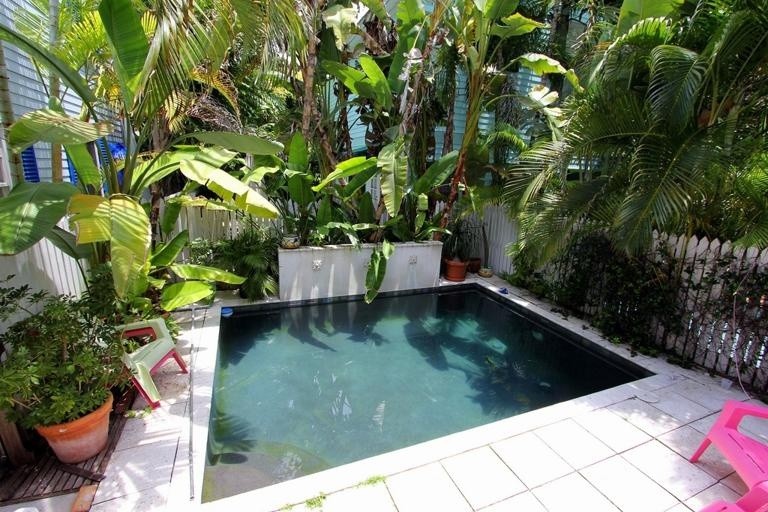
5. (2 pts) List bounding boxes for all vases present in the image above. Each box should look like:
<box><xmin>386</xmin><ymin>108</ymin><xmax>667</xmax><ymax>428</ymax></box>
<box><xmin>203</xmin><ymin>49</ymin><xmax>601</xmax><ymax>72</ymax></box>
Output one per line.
<box><xmin>280</xmin><ymin>234</ymin><xmax>301</xmax><ymax>248</ymax></box>
<box><xmin>468</xmin><ymin>256</ymin><xmax>482</xmax><ymax>274</ymax></box>
<box><xmin>478</xmin><ymin>267</ymin><xmax>493</xmax><ymax>278</ymax></box>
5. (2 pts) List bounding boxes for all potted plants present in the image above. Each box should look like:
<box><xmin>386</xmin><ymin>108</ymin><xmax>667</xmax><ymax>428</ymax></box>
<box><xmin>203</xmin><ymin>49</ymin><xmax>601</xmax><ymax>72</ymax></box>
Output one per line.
<box><xmin>0</xmin><ymin>272</ymin><xmax>150</xmax><ymax>465</ymax></box>
<box><xmin>214</xmin><ymin>243</ymin><xmax>278</xmax><ymax>302</ymax></box>
<box><xmin>445</xmin><ymin>218</ymin><xmax>478</xmax><ymax>282</ymax></box>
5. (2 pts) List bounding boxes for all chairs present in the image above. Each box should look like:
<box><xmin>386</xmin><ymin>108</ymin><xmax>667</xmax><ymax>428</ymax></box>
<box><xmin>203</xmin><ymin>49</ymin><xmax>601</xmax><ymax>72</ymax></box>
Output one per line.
<box><xmin>75</xmin><ymin>316</ymin><xmax>189</xmax><ymax>412</ymax></box>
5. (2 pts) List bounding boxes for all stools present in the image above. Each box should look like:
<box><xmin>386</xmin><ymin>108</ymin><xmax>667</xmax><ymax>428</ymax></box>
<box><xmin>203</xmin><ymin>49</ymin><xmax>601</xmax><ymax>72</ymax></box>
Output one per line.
<box><xmin>698</xmin><ymin>480</ymin><xmax>768</xmax><ymax>511</ymax></box>
<box><xmin>691</xmin><ymin>399</ymin><xmax>767</xmax><ymax>490</ymax></box>
